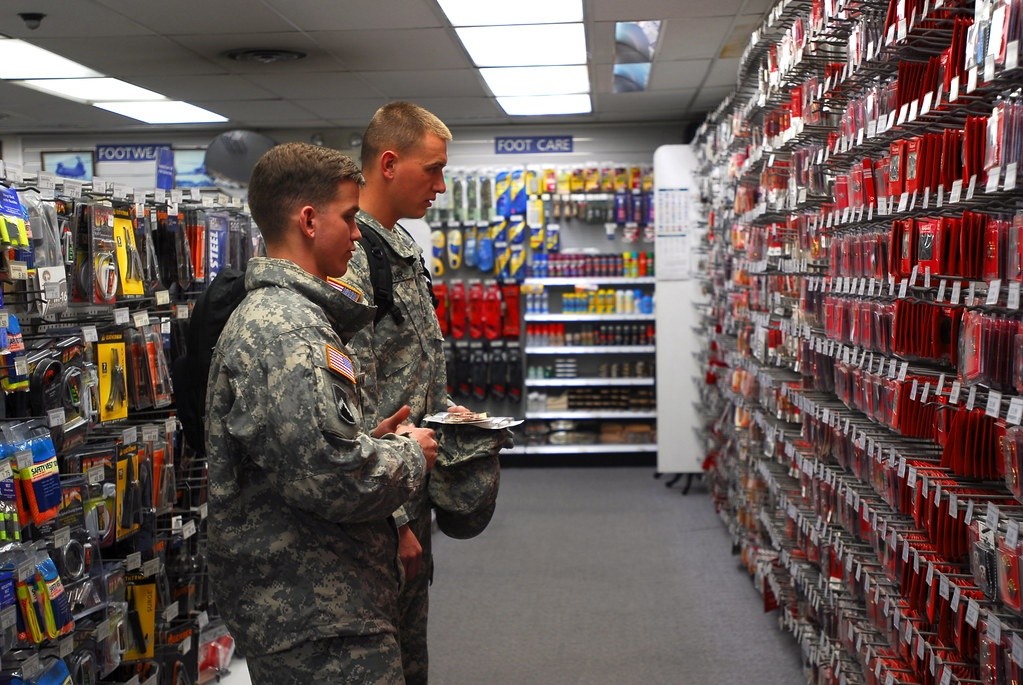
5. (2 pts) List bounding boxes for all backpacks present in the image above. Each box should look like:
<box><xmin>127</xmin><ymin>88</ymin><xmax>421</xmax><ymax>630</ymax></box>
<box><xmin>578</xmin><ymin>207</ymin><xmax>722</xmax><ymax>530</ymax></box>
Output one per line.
<box><xmin>172</xmin><ymin>221</ymin><xmax>440</xmax><ymax>459</ymax></box>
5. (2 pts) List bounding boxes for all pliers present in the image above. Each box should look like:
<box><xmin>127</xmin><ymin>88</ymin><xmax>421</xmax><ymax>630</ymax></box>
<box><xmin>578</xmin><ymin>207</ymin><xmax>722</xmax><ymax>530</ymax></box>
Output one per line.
<box><xmin>180</xmin><ymin>223</ymin><xmax>194</xmax><ymax>279</ymax></box>
<box><xmin>122</xmin><ymin>456</ymin><xmax>141</xmax><ymax>529</ymax></box>
<box><xmin>123</xmin><ymin>226</ymin><xmax>145</xmax><ymax>281</ymax></box>
<box><xmin>105</xmin><ymin>349</ymin><xmax>128</xmax><ymax>411</ymax></box>
<box><xmin>152</xmin><ymin>334</ymin><xmax>175</xmax><ymax>394</ymax></box>
<box><xmin>121</xmin><ymin>584</ymin><xmax>147</xmax><ymax>654</ymax></box>
<box><xmin>136</xmin><ymin>340</ymin><xmax>150</xmax><ymax>397</ymax></box>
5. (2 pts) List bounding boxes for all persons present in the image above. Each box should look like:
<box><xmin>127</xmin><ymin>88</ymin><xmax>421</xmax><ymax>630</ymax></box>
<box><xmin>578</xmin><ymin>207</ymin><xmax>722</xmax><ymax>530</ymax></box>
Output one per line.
<box><xmin>325</xmin><ymin>100</ymin><xmax>478</xmax><ymax>684</ymax></box>
<box><xmin>203</xmin><ymin>144</ymin><xmax>442</xmax><ymax>684</ymax></box>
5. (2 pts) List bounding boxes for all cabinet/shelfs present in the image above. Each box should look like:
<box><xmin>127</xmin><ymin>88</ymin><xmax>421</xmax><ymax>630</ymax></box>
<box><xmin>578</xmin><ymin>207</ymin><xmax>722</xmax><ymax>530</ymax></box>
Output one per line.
<box><xmin>691</xmin><ymin>0</ymin><xmax>1023</xmax><ymax>685</ymax></box>
<box><xmin>0</xmin><ymin>165</ymin><xmax>658</xmax><ymax>685</ymax></box>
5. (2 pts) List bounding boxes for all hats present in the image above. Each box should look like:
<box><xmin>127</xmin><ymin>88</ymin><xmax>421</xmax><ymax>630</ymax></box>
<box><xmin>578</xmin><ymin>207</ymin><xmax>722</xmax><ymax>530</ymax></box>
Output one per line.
<box><xmin>427</xmin><ymin>425</ymin><xmax>500</xmax><ymax>539</ymax></box>
